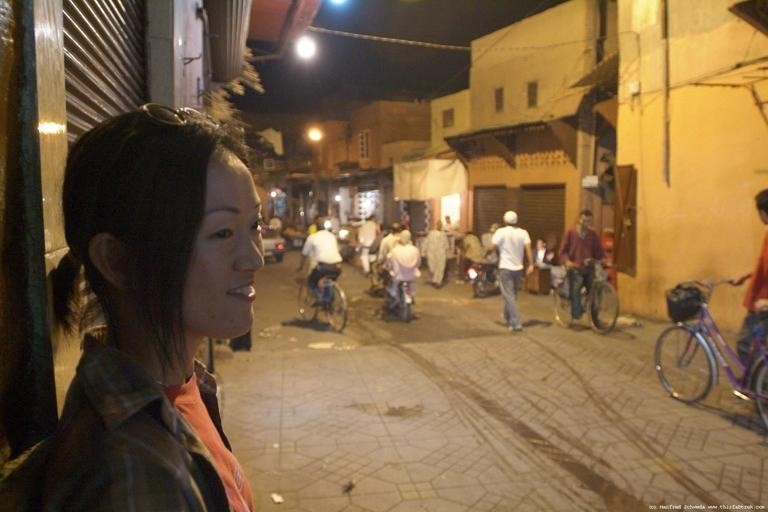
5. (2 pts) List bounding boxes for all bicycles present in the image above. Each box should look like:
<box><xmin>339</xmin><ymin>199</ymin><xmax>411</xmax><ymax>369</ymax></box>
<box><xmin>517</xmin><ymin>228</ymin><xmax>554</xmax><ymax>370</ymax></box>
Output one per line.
<box><xmin>553</xmin><ymin>257</ymin><xmax>619</xmax><ymax>334</ymax></box>
<box><xmin>297</xmin><ymin>267</ymin><xmax>348</xmax><ymax>333</ymax></box>
<box><xmin>369</xmin><ymin>262</ymin><xmax>412</xmax><ymax>321</ymax></box>
<box><xmin>654</xmin><ymin>277</ymin><xmax>767</xmax><ymax>433</ymax></box>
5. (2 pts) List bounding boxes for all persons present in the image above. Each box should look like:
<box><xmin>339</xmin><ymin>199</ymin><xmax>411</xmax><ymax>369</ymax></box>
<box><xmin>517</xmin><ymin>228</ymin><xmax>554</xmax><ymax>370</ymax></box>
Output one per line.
<box><xmin>30</xmin><ymin>103</ymin><xmax>268</xmax><ymax>510</ymax></box>
<box><xmin>294</xmin><ymin>209</ymin><xmax>550</xmax><ymax>332</ymax></box>
<box><xmin>727</xmin><ymin>188</ymin><xmax>767</xmax><ymax>397</ymax></box>
<box><xmin>559</xmin><ymin>208</ymin><xmax>605</xmax><ymax>326</ymax></box>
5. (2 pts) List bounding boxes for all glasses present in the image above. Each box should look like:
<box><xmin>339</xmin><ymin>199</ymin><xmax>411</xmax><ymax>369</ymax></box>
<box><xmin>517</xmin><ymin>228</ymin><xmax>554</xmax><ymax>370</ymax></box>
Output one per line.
<box><xmin>127</xmin><ymin>102</ymin><xmax>202</xmax><ymax>129</ymax></box>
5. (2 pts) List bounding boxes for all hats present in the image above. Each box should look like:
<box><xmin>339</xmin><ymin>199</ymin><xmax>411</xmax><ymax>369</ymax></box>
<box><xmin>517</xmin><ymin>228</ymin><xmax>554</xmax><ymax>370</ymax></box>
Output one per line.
<box><xmin>503</xmin><ymin>212</ymin><xmax>517</xmax><ymax>224</ymax></box>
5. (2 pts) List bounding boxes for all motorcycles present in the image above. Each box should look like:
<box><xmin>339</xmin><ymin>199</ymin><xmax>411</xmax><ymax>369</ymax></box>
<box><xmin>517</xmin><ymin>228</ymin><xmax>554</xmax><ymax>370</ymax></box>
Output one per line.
<box><xmin>469</xmin><ymin>261</ymin><xmax>500</xmax><ymax>299</ymax></box>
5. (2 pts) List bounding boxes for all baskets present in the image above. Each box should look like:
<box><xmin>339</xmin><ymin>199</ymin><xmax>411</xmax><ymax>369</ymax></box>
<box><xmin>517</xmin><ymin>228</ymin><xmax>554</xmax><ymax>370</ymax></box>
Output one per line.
<box><xmin>666</xmin><ymin>287</ymin><xmax>703</xmax><ymax>322</ymax></box>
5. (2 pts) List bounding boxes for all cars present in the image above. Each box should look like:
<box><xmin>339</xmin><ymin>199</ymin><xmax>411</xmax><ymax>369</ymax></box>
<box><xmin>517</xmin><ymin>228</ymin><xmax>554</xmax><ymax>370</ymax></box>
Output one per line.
<box><xmin>259</xmin><ymin>224</ymin><xmax>286</xmax><ymax>263</ymax></box>
